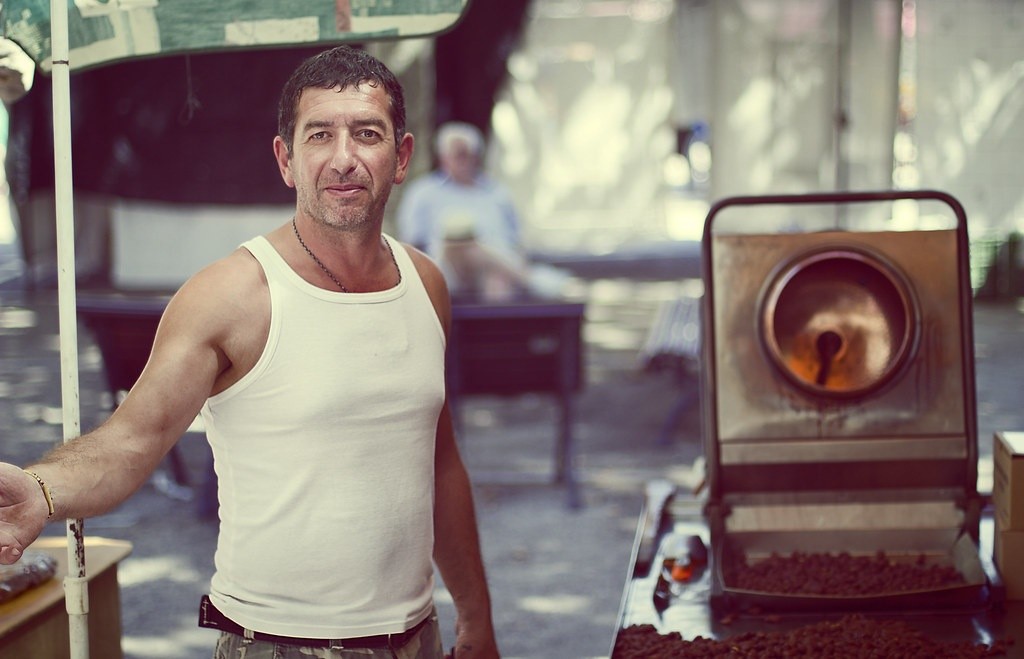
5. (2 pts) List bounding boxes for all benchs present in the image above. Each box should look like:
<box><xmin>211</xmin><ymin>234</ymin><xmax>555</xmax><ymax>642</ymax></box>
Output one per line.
<box><xmin>638</xmin><ymin>297</ymin><xmax>703</xmax><ymax>447</ymax></box>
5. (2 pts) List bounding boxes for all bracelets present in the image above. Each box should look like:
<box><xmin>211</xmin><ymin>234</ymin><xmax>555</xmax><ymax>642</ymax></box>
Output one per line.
<box><xmin>23</xmin><ymin>470</ymin><xmax>55</xmax><ymax>520</ymax></box>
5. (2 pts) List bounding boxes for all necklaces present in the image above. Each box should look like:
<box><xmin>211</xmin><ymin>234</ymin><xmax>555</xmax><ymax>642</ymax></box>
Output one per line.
<box><xmin>293</xmin><ymin>215</ymin><xmax>402</xmax><ymax>292</ymax></box>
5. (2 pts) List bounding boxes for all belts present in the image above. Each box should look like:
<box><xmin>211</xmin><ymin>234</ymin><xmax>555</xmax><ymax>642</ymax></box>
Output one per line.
<box><xmin>199</xmin><ymin>594</ymin><xmax>430</xmax><ymax>650</ymax></box>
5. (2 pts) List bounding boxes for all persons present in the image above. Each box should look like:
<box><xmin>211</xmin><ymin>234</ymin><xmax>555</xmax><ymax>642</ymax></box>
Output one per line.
<box><xmin>0</xmin><ymin>43</ymin><xmax>504</xmax><ymax>659</ymax></box>
<box><xmin>395</xmin><ymin>121</ymin><xmax>524</xmax><ymax>302</ymax></box>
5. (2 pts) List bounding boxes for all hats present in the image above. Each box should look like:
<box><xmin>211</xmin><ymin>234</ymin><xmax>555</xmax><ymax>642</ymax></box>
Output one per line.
<box><xmin>438</xmin><ymin>123</ymin><xmax>483</xmax><ymax>155</ymax></box>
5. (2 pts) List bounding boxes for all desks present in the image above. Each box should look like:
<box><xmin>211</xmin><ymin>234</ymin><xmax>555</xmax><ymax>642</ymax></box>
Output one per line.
<box><xmin>74</xmin><ymin>291</ymin><xmax>588</xmax><ymax>512</ymax></box>
<box><xmin>0</xmin><ymin>535</ymin><xmax>133</xmax><ymax>659</ymax></box>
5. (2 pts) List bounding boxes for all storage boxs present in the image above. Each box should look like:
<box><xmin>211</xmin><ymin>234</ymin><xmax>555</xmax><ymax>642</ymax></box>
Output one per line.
<box><xmin>992</xmin><ymin>431</ymin><xmax>1024</xmax><ymax>603</ymax></box>
